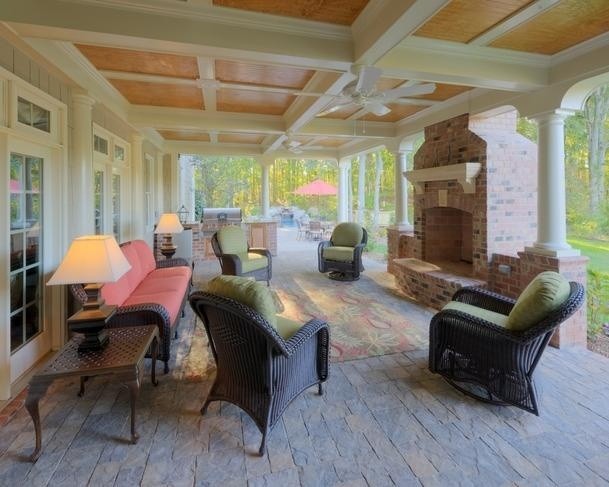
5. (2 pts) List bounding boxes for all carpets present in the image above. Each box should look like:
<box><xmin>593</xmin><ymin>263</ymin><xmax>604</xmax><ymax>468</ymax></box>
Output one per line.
<box><xmin>171</xmin><ymin>272</ymin><xmax>431</xmax><ymax>384</ymax></box>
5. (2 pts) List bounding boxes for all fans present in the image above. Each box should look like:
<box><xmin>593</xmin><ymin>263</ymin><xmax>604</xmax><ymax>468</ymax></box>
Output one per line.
<box><xmin>293</xmin><ymin>64</ymin><xmax>437</xmax><ymax>118</ymax></box>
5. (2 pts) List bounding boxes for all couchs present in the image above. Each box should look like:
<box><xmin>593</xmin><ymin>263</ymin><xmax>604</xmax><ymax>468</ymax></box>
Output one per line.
<box><xmin>68</xmin><ymin>239</ymin><xmax>195</xmax><ymax>375</ymax></box>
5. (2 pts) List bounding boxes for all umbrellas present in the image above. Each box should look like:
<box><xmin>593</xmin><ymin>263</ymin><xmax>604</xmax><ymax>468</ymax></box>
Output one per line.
<box><xmin>291</xmin><ymin>177</ymin><xmax>338</xmax><ymax>221</ymax></box>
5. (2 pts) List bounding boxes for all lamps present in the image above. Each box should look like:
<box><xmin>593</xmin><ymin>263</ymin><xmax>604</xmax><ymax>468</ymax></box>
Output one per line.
<box><xmin>153</xmin><ymin>214</ymin><xmax>184</xmax><ymax>250</ymax></box>
<box><xmin>45</xmin><ymin>234</ymin><xmax>133</xmax><ymax>354</ymax></box>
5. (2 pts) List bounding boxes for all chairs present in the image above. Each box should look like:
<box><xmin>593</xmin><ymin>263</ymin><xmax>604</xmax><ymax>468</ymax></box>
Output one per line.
<box><xmin>295</xmin><ymin>218</ymin><xmax>310</xmax><ymax>241</ymax></box>
<box><xmin>308</xmin><ymin>221</ymin><xmax>322</xmax><ymax>241</ymax></box>
<box><xmin>317</xmin><ymin>222</ymin><xmax>369</xmax><ymax>282</ymax></box>
<box><xmin>186</xmin><ymin>274</ymin><xmax>331</xmax><ymax>458</ymax></box>
<box><xmin>210</xmin><ymin>223</ymin><xmax>273</xmax><ymax>286</ymax></box>
<box><xmin>425</xmin><ymin>271</ymin><xmax>586</xmax><ymax>419</ymax></box>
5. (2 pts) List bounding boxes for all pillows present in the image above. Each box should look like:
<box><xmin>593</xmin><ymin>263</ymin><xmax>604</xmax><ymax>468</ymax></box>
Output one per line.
<box><xmin>506</xmin><ymin>270</ymin><xmax>571</xmax><ymax>331</ymax></box>
<box><xmin>205</xmin><ymin>272</ymin><xmax>279</xmax><ymax>336</ymax></box>
<box><xmin>216</xmin><ymin>224</ymin><xmax>249</xmax><ymax>254</ymax></box>
<box><xmin>331</xmin><ymin>222</ymin><xmax>364</xmax><ymax>247</ymax></box>
